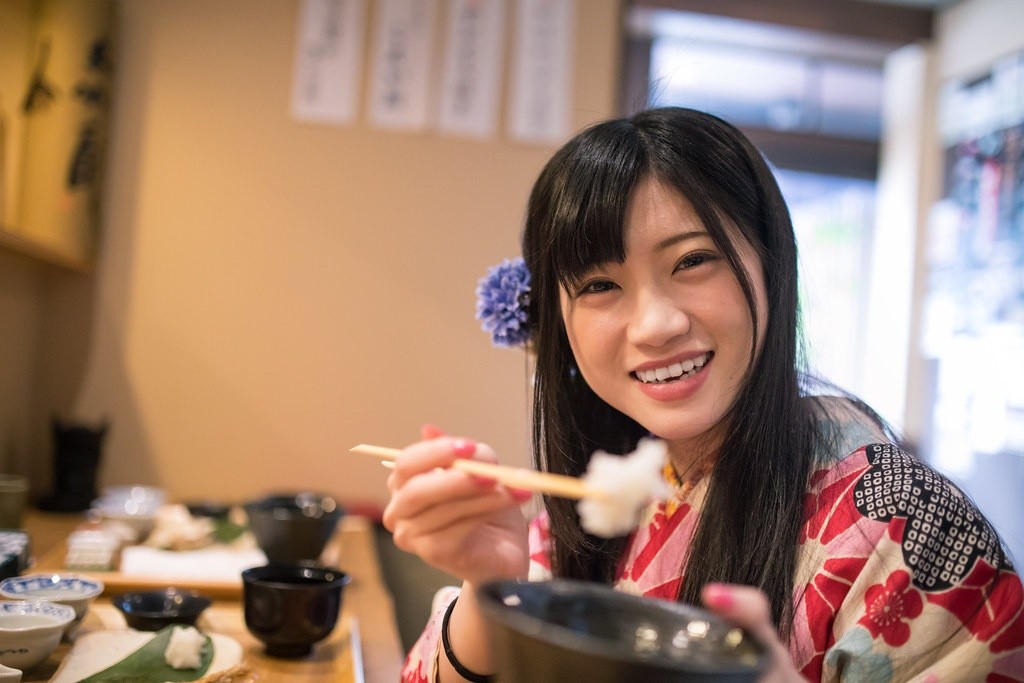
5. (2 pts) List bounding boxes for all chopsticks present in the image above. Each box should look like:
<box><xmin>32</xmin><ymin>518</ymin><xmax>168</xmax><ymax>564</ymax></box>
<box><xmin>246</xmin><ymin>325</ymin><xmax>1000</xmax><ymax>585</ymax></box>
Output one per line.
<box><xmin>351</xmin><ymin>442</ymin><xmax>588</xmax><ymax>501</ymax></box>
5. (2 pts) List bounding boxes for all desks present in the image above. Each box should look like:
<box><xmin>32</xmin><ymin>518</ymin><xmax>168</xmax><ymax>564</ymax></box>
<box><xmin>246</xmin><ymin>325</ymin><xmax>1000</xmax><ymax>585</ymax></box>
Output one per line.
<box><xmin>24</xmin><ymin>512</ymin><xmax>405</xmax><ymax>683</ymax></box>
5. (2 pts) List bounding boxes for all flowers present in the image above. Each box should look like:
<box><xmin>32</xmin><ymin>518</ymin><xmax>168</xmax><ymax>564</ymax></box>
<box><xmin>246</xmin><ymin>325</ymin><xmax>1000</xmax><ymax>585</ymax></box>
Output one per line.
<box><xmin>473</xmin><ymin>258</ymin><xmax>534</xmax><ymax>348</ymax></box>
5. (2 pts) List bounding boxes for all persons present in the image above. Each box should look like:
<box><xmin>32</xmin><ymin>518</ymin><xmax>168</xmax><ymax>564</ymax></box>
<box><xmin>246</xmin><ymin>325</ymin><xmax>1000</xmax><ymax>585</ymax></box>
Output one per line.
<box><xmin>382</xmin><ymin>106</ymin><xmax>1024</xmax><ymax>683</ymax></box>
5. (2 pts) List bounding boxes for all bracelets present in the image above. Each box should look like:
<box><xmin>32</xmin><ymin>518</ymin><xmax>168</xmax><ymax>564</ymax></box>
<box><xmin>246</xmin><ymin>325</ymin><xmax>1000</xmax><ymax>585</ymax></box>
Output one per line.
<box><xmin>438</xmin><ymin>596</ymin><xmax>501</xmax><ymax>683</ymax></box>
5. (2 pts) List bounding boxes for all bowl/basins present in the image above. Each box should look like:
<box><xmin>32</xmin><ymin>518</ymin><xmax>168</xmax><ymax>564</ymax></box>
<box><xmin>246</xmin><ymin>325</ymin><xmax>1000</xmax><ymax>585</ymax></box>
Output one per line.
<box><xmin>241</xmin><ymin>563</ymin><xmax>351</xmax><ymax>658</ymax></box>
<box><xmin>0</xmin><ymin>601</ymin><xmax>77</xmax><ymax>675</ymax></box>
<box><xmin>110</xmin><ymin>592</ymin><xmax>213</xmax><ymax>632</ymax></box>
<box><xmin>243</xmin><ymin>495</ymin><xmax>344</xmax><ymax>569</ymax></box>
<box><xmin>0</xmin><ymin>573</ymin><xmax>105</xmax><ymax>636</ymax></box>
<box><xmin>471</xmin><ymin>578</ymin><xmax>772</xmax><ymax>683</ymax></box>
